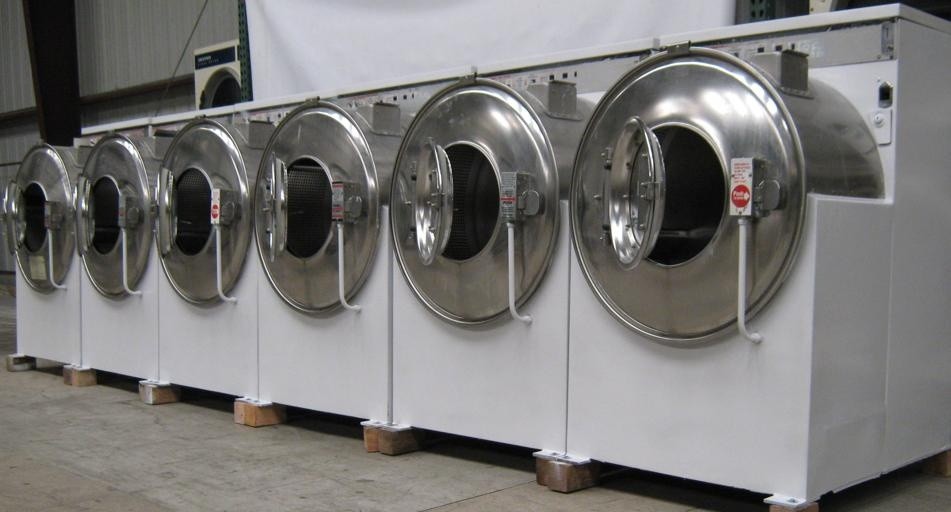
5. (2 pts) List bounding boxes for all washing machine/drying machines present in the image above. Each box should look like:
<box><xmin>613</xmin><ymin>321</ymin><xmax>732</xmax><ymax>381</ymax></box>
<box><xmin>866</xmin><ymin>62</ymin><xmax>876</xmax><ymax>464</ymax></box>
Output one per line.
<box><xmin>0</xmin><ymin>1</ymin><xmax>951</xmax><ymax>512</ymax></box>
